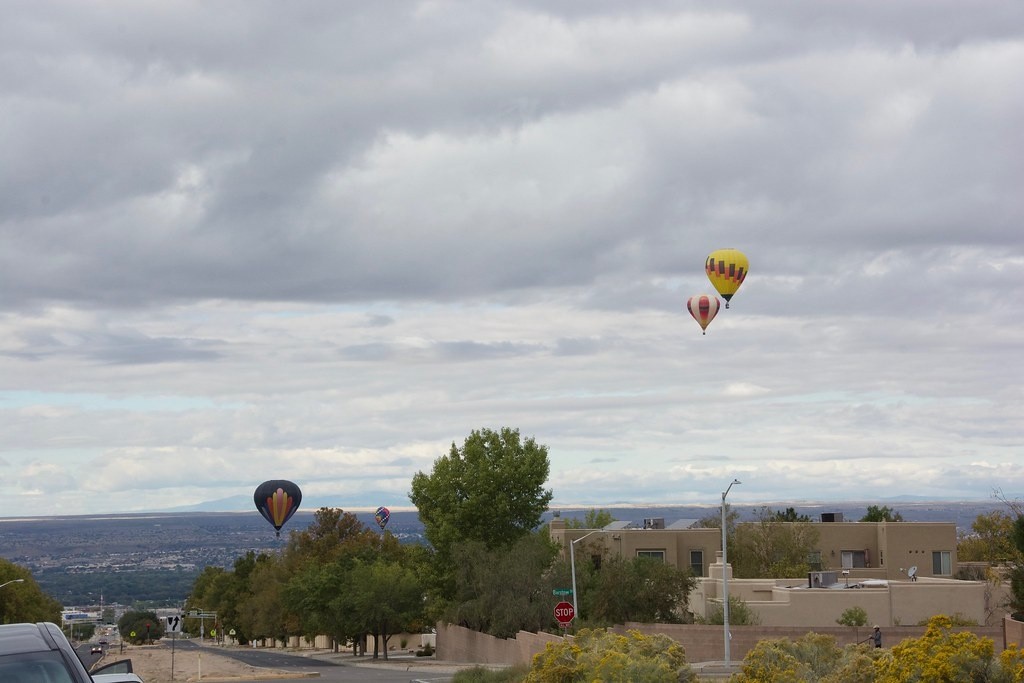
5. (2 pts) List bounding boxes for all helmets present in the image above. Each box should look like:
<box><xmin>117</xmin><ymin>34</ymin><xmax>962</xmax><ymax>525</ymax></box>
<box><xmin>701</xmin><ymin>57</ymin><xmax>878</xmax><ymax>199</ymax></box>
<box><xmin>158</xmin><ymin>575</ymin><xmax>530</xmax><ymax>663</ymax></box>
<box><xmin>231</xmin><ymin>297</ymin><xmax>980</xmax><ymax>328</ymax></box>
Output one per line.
<box><xmin>873</xmin><ymin>625</ymin><xmax>879</xmax><ymax>629</ymax></box>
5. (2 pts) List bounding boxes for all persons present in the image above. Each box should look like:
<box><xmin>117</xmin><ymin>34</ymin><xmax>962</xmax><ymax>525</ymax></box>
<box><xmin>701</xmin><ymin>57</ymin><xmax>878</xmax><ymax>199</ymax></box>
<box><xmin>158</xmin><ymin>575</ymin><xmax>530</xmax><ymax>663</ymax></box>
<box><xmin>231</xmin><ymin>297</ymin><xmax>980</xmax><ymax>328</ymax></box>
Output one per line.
<box><xmin>868</xmin><ymin>625</ymin><xmax>881</xmax><ymax>648</ymax></box>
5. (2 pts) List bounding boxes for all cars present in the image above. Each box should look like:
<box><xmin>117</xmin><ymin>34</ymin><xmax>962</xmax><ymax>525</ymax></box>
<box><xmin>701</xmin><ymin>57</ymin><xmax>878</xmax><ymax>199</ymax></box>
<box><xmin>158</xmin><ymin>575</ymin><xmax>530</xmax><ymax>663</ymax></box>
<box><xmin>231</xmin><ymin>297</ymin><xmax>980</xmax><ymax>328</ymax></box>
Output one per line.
<box><xmin>89</xmin><ymin>644</ymin><xmax>104</xmax><ymax>655</ymax></box>
<box><xmin>0</xmin><ymin>621</ymin><xmax>144</xmax><ymax>683</ymax></box>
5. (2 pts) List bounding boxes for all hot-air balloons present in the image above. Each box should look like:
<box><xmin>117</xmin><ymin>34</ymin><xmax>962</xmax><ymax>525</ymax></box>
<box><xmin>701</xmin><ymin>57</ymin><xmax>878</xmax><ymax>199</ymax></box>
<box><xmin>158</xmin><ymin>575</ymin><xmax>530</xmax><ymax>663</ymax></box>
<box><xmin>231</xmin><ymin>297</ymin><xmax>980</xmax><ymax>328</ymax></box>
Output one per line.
<box><xmin>372</xmin><ymin>507</ymin><xmax>390</xmax><ymax>529</ymax></box>
<box><xmin>253</xmin><ymin>481</ymin><xmax>303</xmax><ymax>538</ymax></box>
<box><xmin>687</xmin><ymin>295</ymin><xmax>720</xmax><ymax>335</ymax></box>
<box><xmin>705</xmin><ymin>248</ymin><xmax>749</xmax><ymax>310</ymax></box>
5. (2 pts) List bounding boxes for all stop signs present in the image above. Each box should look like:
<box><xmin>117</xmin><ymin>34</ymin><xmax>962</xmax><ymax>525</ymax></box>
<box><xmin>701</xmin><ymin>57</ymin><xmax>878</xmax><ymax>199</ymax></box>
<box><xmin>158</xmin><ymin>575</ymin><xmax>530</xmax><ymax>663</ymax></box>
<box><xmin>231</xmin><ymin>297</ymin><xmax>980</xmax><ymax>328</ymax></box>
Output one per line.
<box><xmin>554</xmin><ymin>601</ymin><xmax>574</xmax><ymax>622</ymax></box>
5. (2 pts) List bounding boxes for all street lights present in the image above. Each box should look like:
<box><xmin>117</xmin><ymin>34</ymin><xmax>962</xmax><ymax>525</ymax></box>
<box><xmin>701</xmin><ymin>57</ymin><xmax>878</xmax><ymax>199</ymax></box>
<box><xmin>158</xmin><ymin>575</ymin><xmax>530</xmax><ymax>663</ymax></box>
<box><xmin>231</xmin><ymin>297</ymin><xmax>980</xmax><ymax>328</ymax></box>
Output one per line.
<box><xmin>192</xmin><ymin>607</ymin><xmax>204</xmax><ymax>643</ymax></box>
<box><xmin>570</xmin><ymin>531</ymin><xmax>610</xmax><ymax>618</ymax></box>
<box><xmin>722</xmin><ymin>479</ymin><xmax>742</xmax><ymax>667</ymax></box>
